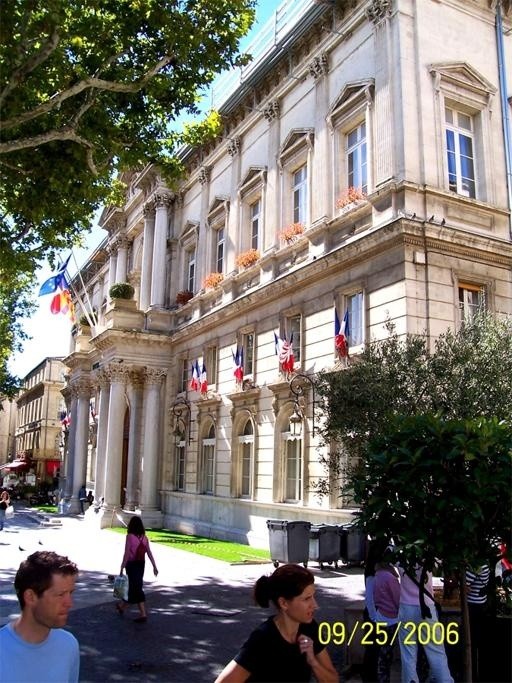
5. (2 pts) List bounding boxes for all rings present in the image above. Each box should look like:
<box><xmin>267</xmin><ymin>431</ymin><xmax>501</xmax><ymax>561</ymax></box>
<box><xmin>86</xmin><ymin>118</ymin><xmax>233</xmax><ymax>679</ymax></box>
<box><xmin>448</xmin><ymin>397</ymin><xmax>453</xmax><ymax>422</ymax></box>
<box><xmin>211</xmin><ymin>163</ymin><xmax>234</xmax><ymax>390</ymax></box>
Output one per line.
<box><xmin>304</xmin><ymin>638</ymin><xmax>307</xmax><ymax>642</ymax></box>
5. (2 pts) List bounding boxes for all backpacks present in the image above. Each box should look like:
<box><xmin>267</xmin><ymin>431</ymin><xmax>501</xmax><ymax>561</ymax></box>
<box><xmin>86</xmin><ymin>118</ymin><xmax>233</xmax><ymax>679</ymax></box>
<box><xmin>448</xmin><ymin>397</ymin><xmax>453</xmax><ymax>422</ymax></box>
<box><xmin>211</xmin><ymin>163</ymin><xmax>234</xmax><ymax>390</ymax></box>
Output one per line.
<box><xmin>132</xmin><ymin>531</ymin><xmax>147</xmax><ymax>564</ymax></box>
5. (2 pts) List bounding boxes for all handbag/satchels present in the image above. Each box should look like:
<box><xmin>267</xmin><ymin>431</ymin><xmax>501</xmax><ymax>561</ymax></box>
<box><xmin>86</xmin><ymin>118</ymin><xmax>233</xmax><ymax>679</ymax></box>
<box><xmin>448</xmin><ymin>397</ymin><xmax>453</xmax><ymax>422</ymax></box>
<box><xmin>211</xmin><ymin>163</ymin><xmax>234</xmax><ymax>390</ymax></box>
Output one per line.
<box><xmin>113</xmin><ymin>573</ymin><xmax>130</xmax><ymax>601</ymax></box>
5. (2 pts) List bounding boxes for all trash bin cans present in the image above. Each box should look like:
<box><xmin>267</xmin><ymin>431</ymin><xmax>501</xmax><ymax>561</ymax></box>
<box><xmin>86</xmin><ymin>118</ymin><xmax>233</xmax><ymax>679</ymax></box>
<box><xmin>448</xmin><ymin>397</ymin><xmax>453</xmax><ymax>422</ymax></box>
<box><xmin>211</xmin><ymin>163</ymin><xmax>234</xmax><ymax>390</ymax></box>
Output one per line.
<box><xmin>266</xmin><ymin>519</ymin><xmax>311</xmax><ymax>568</ymax></box>
<box><xmin>340</xmin><ymin>523</ymin><xmax>367</xmax><ymax>567</ymax></box>
<box><xmin>309</xmin><ymin>523</ymin><xmax>343</xmax><ymax>570</ymax></box>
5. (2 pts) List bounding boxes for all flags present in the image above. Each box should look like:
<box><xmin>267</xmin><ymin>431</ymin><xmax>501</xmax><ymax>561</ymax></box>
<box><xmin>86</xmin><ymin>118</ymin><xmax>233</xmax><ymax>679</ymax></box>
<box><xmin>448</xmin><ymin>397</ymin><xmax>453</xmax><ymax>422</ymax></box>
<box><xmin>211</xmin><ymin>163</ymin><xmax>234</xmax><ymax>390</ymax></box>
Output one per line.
<box><xmin>39</xmin><ymin>250</ymin><xmax>79</xmax><ymax>327</ymax></box>
<box><xmin>185</xmin><ymin>302</ymin><xmax>350</xmax><ymax>393</ymax></box>
<box><xmin>60</xmin><ymin>410</ymin><xmax>72</xmax><ymax>426</ymax></box>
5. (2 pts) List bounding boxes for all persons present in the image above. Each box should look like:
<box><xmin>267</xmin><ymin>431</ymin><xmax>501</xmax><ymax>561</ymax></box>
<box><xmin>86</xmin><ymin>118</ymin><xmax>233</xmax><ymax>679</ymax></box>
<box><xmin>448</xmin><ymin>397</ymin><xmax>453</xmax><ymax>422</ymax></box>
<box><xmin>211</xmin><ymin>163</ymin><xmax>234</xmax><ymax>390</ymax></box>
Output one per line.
<box><xmin>94</xmin><ymin>496</ymin><xmax>105</xmax><ymax>513</ymax></box>
<box><xmin>79</xmin><ymin>483</ymin><xmax>90</xmax><ymax>515</ymax></box>
<box><xmin>361</xmin><ymin>534</ymin><xmax>511</xmax><ymax>683</ymax></box>
<box><xmin>116</xmin><ymin>515</ymin><xmax>158</xmax><ymax>621</ymax></box>
<box><xmin>87</xmin><ymin>489</ymin><xmax>95</xmax><ymax>506</ymax></box>
<box><xmin>1</xmin><ymin>550</ymin><xmax>80</xmax><ymax>682</ymax></box>
<box><xmin>1</xmin><ymin>491</ymin><xmax>12</xmax><ymax>532</ymax></box>
<box><xmin>211</xmin><ymin>565</ymin><xmax>340</xmax><ymax>682</ymax></box>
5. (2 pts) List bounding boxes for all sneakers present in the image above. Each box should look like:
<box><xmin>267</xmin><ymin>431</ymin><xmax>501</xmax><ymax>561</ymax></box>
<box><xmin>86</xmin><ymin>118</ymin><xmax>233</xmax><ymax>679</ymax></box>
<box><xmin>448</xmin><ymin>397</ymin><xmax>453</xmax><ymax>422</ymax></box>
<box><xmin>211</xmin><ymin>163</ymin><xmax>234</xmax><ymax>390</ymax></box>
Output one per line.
<box><xmin>114</xmin><ymin>602</ymin><xmax>149</xmax><ymax>625</ymax></box>
